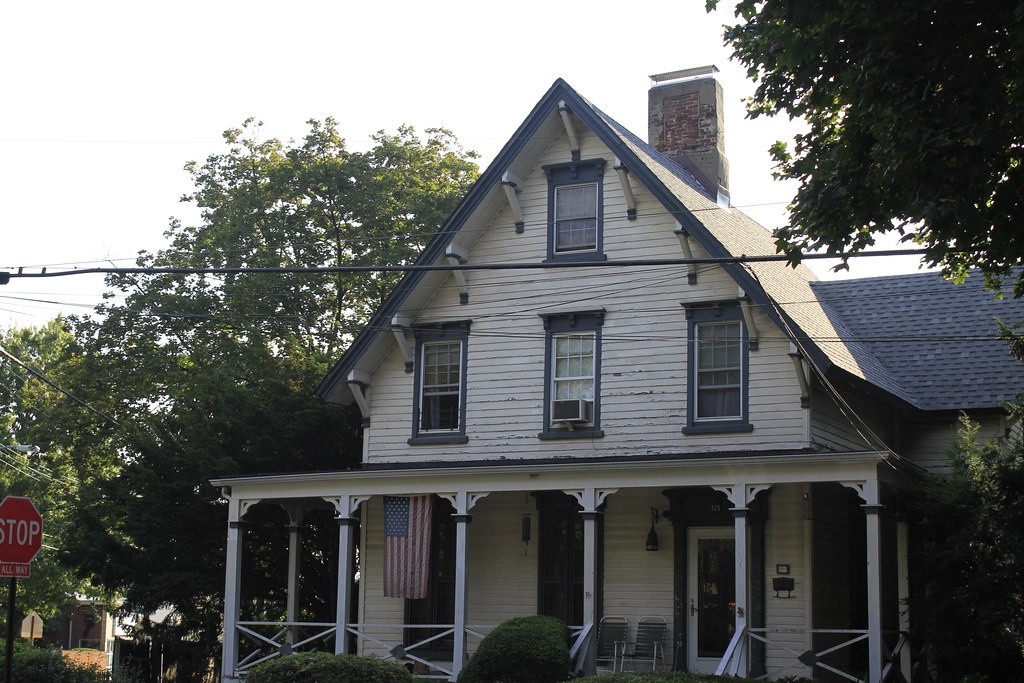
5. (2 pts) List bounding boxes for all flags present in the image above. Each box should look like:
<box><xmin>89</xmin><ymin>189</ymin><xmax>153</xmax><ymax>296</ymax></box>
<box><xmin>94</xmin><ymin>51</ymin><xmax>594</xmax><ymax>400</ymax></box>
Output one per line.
<box><xmin>381</xmin><ymin>494</ymin><xmax>433</xmax><ymax>601</ymax></box>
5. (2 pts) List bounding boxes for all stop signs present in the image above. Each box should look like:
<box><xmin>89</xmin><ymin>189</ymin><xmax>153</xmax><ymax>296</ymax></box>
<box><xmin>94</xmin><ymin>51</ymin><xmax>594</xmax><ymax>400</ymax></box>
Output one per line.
<box><xmin>0</xmin><ymin>496</ymin><xmax>44</xmax><ymax>564</ymax></box>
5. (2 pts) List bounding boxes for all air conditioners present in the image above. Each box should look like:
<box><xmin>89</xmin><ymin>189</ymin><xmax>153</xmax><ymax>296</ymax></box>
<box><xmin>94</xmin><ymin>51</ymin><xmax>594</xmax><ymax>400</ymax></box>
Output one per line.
<box><xmin>551</xmin><ymin>399</ymin><xmax>589</xmax><ymax>423</ymax></box>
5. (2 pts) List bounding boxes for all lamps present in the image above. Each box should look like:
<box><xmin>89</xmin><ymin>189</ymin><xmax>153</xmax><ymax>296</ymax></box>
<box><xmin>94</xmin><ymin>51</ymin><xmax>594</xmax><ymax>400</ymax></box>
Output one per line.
<box><xmin>645</xmin><ymin>508</ymin><xmax>659</xmax><ymax>551</ymax></box>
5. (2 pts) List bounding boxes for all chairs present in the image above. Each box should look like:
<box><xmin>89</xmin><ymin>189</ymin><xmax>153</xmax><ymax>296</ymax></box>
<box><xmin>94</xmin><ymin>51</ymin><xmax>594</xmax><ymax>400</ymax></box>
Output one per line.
<box><xmin>597</xmin><ymin>616</ymin><xmax>666</xmax><ymax>673</ymax></box>
